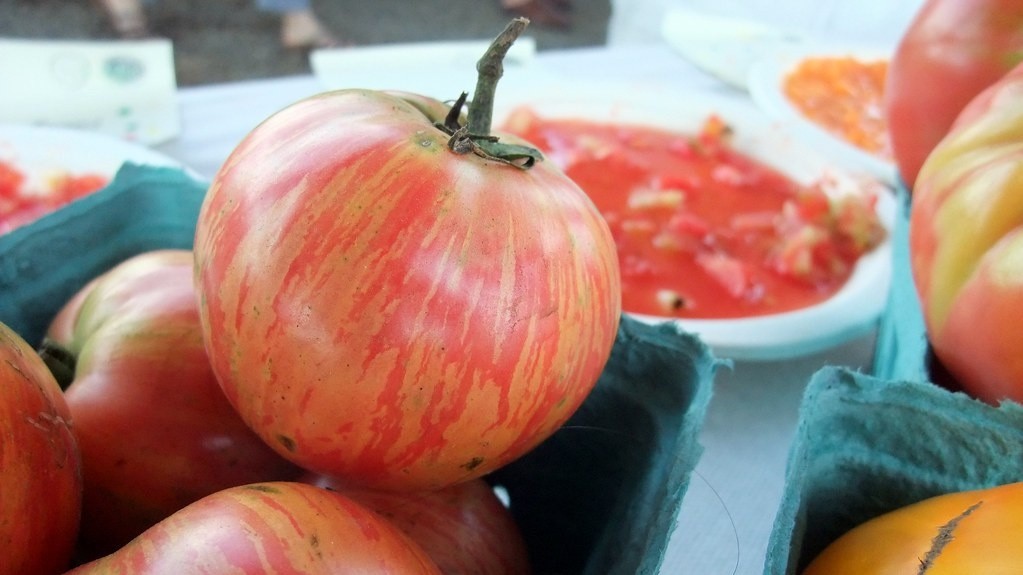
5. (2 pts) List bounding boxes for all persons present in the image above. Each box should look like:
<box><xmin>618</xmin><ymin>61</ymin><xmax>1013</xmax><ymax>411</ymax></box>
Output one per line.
<box><xmin>97</xmin><ymin>0</ymin><xmax>356</xmax><ymax>49</ymax></box>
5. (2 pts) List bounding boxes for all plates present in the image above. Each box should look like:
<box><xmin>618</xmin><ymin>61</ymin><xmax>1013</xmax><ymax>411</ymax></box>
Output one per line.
<box><xmin>493</xmin><ymin>79</ymin><xmax>894</xmax><ymax>362</ymax></box>
<box><xmin>0</xmin><ymin>124</ymin><xmax>204</xmax><ymax>236</ymax></box>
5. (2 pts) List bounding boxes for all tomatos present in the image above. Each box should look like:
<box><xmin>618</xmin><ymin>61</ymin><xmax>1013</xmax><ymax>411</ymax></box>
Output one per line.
<box><xmin>0</xmin><ymin>0</ymin><xmax>1023</xmax><ymax>575</ymax></box>
